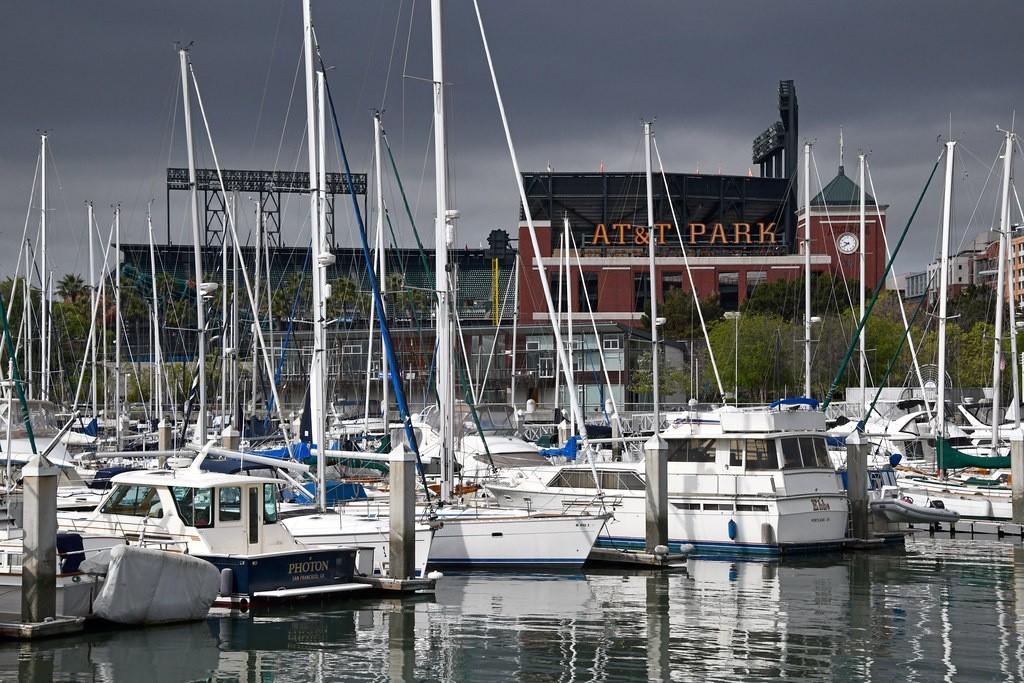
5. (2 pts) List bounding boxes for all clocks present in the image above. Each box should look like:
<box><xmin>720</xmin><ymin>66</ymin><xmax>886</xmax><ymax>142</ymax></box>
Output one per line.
<box><xmin>836</xmin><ymin>232</ymin><xmax>859</xmax><ymax>255</ymax></box>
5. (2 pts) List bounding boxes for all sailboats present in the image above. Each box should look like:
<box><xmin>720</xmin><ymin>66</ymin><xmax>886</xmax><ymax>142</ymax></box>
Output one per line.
<box><xmin>1</xmin><ymin>0</ymin><xmax>1024</xmax><ymax>640</ymax></box>
